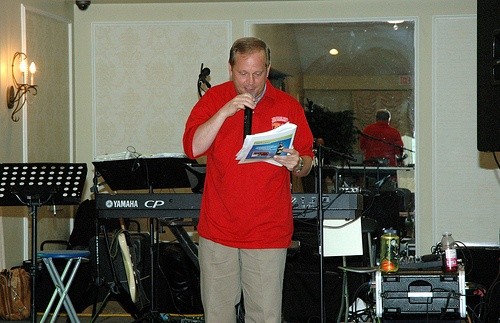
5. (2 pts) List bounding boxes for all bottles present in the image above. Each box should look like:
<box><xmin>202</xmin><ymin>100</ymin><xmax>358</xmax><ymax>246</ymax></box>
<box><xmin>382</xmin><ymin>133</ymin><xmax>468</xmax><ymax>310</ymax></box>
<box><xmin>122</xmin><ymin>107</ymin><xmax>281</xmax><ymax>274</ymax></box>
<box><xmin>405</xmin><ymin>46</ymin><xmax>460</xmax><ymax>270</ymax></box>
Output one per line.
<box><xmin>442</xmin><ymin>232</ymin><xmax>454</xmax><ymax>250</ymax></box>
<box><xmin>381</xmin><ymin>230</ymin><xmax>399</xmax><ymax>272</ymax></box>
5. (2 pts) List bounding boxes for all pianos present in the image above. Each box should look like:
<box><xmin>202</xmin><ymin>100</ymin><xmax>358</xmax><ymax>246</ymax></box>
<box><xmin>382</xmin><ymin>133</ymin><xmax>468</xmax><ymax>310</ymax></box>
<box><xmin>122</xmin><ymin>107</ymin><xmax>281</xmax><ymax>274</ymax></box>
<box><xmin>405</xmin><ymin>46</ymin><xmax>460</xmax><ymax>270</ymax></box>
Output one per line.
<box><xmin>315</xmin><ymin>165</ymin><xmax>415</xmax><ymax>188</ymax></box>
<box><xmin>91</xmin><ymin>192</ymin><xmax>364</xmax><ymax>323</ymax></box>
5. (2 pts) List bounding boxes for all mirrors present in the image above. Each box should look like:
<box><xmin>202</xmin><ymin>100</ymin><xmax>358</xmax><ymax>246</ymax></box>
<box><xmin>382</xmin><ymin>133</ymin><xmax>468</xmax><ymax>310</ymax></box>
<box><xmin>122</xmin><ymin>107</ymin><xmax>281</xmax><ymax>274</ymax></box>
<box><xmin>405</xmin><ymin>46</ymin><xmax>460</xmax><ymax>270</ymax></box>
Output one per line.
<box><xmin>246</xmin><ymin>16</ymin><xmax>419</xmax><ymax>260</ymax></box>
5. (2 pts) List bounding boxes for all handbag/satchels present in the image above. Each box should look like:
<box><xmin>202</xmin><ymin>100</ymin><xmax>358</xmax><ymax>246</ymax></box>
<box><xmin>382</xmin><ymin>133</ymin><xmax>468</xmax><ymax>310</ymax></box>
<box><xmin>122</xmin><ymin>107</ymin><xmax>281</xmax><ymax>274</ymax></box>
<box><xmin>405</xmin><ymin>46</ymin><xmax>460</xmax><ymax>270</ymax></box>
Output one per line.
<box><xmin>0</xmin><ymin>268</ymin><xmax>32</xmax><ymax>320</ymax></box>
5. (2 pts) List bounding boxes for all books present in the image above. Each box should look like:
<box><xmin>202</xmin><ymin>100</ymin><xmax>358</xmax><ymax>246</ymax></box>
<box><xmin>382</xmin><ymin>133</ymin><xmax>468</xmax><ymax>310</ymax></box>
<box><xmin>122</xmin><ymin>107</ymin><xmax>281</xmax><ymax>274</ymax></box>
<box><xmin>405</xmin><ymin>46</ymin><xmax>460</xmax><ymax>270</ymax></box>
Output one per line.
<box><xmin>235</xmin><ymin>122</ymin><xmax>297</xmax><ymax>167</ymax></box>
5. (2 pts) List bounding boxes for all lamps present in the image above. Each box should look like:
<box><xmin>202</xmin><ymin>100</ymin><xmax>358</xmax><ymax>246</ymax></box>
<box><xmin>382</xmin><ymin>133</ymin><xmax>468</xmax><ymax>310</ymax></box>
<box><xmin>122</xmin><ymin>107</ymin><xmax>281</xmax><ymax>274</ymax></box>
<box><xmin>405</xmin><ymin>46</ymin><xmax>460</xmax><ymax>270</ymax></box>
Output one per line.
<box><xmin>6</xmin><ymin>52</ymin><xmax>38</xmax><ymax>122</ymax></box>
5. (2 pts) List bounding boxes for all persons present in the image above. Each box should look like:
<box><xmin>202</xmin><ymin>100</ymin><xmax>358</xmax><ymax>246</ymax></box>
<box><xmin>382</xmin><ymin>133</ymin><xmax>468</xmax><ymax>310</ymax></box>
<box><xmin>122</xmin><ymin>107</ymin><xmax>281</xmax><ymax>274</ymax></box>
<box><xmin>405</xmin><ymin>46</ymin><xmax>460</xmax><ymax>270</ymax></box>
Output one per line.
<box><xmin>360</xmin><ymin>109</ymin><xmax>404</xmax><ymax>187</ymax></box>
<box><xmin>183</xmin><ymin>36</ymin><xmax>314</xmax><ymax>323</ymax></box>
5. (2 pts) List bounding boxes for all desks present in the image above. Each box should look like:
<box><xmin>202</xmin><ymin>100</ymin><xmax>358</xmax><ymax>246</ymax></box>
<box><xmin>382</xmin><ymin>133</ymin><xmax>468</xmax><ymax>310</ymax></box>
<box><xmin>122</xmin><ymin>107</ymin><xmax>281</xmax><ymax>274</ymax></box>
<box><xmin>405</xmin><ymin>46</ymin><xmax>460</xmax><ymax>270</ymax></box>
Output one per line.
<box><xmin>324</xmin><ymin>166</ymin><xmax>414</xmax><ymax>193</ymax></box>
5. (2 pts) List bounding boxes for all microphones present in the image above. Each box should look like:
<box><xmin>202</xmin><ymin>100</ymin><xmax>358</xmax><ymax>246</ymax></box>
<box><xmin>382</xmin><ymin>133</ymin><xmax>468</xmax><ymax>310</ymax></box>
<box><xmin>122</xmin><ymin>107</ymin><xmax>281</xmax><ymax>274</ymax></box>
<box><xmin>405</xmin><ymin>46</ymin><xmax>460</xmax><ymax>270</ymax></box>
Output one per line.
<box><xmin>199</xmin><ymin>68</ymin><xmax>210</xmax><ymax>87</ymax></box>
<box><xmin>244</xmin><ymin>106</ymin><xmax>252</xmax><ymax>139</ymax></box>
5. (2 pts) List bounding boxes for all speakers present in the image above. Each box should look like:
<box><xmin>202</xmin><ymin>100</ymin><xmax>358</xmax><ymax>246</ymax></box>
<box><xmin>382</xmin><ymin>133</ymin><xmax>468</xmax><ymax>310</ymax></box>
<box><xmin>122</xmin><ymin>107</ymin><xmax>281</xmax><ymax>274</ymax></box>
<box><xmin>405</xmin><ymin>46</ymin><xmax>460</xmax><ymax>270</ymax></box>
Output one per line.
<box><xmin>477</xmin><ymin>0</ymin><xmax>500</xmax><ymax>151</ymax></box>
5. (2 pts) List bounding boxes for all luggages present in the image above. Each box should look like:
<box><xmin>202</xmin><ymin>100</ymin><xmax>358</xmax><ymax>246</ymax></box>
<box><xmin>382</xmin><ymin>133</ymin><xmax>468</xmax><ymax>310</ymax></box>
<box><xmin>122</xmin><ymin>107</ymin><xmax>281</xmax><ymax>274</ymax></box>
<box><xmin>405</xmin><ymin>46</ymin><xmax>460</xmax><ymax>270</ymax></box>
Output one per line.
<box><xmin>91</xmin><ymin>228</ymin><xmax>151</xmax><ymax>299</ymax></box>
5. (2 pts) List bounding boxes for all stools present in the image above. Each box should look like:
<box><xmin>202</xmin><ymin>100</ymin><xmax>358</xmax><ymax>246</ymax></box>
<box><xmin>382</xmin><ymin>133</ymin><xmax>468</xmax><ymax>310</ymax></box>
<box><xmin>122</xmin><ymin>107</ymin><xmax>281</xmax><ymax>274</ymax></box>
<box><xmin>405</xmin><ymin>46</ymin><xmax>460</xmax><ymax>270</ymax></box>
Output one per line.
<box><xmin>36</xmin><ymin>250</ymin><xmax>90</xmax><ymax>323</ymax></box>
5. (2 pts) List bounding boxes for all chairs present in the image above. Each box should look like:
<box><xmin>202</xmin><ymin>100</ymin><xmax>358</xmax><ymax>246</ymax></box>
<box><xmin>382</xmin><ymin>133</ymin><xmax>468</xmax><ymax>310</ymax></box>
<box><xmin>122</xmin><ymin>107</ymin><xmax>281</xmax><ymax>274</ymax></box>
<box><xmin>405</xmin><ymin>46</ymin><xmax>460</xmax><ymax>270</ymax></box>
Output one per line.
<box><xmin>336</xmin><ymin>218</ymin><xmax>381</xmax><ymax>323</ymax></box>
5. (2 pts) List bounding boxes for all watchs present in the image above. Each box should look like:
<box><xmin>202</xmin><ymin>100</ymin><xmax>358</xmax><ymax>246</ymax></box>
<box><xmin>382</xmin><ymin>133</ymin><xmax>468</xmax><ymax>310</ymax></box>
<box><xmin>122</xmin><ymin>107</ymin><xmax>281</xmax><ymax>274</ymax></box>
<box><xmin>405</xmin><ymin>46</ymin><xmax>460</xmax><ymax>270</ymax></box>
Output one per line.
<box><xmin>292</xmin><ymin>156</ymin><xmax>304</xmax><ymax>172</ymax></box>
